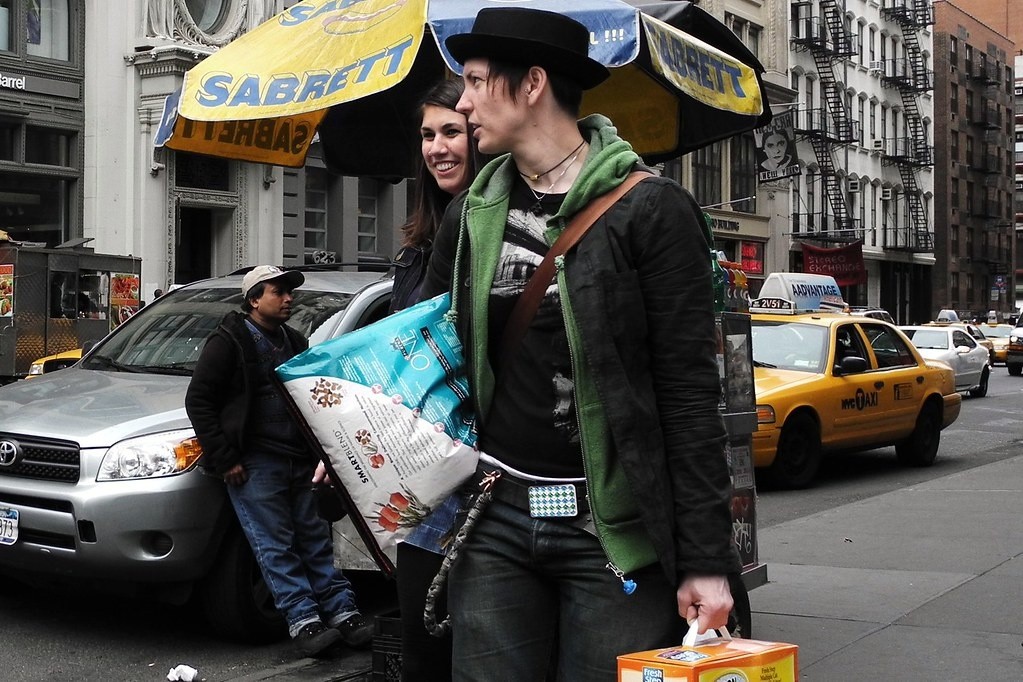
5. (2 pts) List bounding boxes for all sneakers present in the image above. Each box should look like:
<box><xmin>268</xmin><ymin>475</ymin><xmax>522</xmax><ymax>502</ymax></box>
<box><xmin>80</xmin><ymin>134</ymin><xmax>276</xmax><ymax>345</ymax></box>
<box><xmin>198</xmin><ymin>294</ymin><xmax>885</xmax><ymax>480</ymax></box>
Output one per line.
<box><xmin>292</xmin><ymin>622</ymin><xmax>342</xmax><ymax>650</ymax></box>
<box><xmin>336</xmin><ymin>615</ymin><xmax>375</xmax><ymax>643</ymax></box>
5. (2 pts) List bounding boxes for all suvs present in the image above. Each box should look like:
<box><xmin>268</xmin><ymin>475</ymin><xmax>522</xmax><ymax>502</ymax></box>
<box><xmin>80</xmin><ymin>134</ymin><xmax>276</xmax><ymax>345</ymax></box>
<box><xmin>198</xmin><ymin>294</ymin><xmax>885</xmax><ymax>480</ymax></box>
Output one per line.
<box><xmin>1</xmin><ymin>262</ymin><xmax>402</xmax><ymax>653</ymax></box>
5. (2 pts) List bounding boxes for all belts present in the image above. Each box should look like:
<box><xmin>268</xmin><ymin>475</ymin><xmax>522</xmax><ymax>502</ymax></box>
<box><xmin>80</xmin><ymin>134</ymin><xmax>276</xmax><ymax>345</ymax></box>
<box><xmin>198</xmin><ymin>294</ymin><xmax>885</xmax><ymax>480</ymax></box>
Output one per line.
<box><xmin>470</xmin><ymin>466</ymin><xmax>589</xmax><ymax>521</ymax></box>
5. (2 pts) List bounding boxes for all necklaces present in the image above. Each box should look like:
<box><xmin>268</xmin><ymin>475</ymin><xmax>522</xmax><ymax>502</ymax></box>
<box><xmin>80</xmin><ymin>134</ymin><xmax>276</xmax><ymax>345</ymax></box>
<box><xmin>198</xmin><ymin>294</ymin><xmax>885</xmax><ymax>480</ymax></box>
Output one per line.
<box><xmin>518</xmin><ymin>139</ymin><xmax>588</xmax><ymax>216</ymax></box>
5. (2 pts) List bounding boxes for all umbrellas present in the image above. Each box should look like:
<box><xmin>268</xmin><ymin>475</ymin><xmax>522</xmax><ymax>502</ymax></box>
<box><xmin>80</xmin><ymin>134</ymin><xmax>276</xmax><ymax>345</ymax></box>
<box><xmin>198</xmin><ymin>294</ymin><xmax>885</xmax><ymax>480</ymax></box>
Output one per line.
<box><xmin>153</xmin><ymin>0</ymin><xmax>772</xmax><ymax>178</ymax></box>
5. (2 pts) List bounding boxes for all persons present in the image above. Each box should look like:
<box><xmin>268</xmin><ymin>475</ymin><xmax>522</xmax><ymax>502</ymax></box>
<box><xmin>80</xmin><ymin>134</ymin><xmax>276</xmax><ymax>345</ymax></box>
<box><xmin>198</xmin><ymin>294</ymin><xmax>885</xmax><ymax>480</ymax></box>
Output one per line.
<box><xmin>414</xmin><ymin>7</ymin><xmax>744</xmax><ymax>682</ymax></box>
<box><xmin>52</xmin><ymin>273</ymin><xmax>68</xmax><ymax>318</ymax></box>
<box><xmin>184</xmin><ymin>265</ymin><xmax>377</xmax><ymax>658</ymax></box>
<box><xmin>761</xmin><ymin>122</ymin><xmax>799</xmax><ymax>173</ymax></box>
<box><xmin>313</xmin><ymin>76</ymin><xmax>493</xmax><ymax>682</ymax></box>
<box><xmin>786</xmin><ymin>332</ymin><xmax>846</xmax><ymax>366</ymax></box>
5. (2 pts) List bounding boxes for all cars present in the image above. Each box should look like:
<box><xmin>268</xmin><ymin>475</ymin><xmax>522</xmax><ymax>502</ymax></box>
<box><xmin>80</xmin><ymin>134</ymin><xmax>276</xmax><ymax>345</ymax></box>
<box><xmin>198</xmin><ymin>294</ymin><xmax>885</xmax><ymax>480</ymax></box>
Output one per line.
<box><xmin>20</xmin><ymin>348</ymin><xmax>88</xmax><ymax>381</ymax></box>
<box><xmin>895</xmin><ymin>326</ymin><xmax>992</xmax><ymax>398</ymax></box>
<box><xmin>741</xmin><ymin>272</ymin><xmax>963</xmax><ymax>491</ymax></box>
<box><xmin>970</xmin><ymin>309</ymin><xmax>1023</xmax><ymax>378</ymax></box>
<box><xmin>921</xmin><ymin>309</ymin><xmax>996</xmax><ymax>371</ymax></box>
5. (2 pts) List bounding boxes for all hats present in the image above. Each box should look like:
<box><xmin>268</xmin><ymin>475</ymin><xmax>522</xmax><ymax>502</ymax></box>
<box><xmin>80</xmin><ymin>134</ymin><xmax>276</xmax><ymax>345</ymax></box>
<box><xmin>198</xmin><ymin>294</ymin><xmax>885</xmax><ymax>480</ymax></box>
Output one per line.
<box><xmin>241</xmin><ymin>265</ymin><xmax>306</xmax><ymax>299</ymax></box>
<box><xmin>445</xmin><ymin>7</ymin><xmax>612</xmax><ymax>90</ymax></box>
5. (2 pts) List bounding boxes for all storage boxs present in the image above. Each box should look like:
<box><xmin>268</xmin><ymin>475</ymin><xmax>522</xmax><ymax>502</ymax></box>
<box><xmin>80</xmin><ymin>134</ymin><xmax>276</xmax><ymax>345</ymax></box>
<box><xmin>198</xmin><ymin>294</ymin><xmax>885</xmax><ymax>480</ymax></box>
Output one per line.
<box><xmin>617</xmin><ymin>638</ymin><xmax>799</xmax><ymax>682</ymax></box>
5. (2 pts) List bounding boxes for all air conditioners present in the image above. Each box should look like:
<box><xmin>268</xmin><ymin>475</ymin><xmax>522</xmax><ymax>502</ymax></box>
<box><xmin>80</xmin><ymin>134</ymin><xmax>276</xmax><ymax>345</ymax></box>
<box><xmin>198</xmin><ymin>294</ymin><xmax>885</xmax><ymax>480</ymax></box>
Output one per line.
<box><xmin>871</xmin><ymin>138</ymin><xmax>886</xmax><ymax>150</ymax></box>
<box><xmin>870</xmin><ymin>60</ymin><xmax>885</xmax><ymax>72</ymax></box>
<box><xmin>1015</xmin><ymin>89</ymin><xmax>1022</xmax><ymax>95</ymax></box>
<box><xmin>882</xmin><ymin>189</ymin><xmax>892</xmax><ymax>201</ymax></box>
<box><xmin>848</xmin><ymin>180</ymin><xmax>860</xmax><ymax>192</ymax></box>
<box><xmin>1016</xmin><ymin>183</ymin><xmax>1023</xmax><ymax>189</ymax></box>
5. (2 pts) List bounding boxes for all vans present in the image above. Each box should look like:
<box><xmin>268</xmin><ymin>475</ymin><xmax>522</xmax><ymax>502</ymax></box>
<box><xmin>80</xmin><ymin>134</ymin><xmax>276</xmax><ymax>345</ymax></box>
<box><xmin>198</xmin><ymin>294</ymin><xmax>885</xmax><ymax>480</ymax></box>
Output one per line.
<box><xmin>845</xmin><ymin>306</ymin><xmax>897</xmax><ymax>327</ymax></box>
